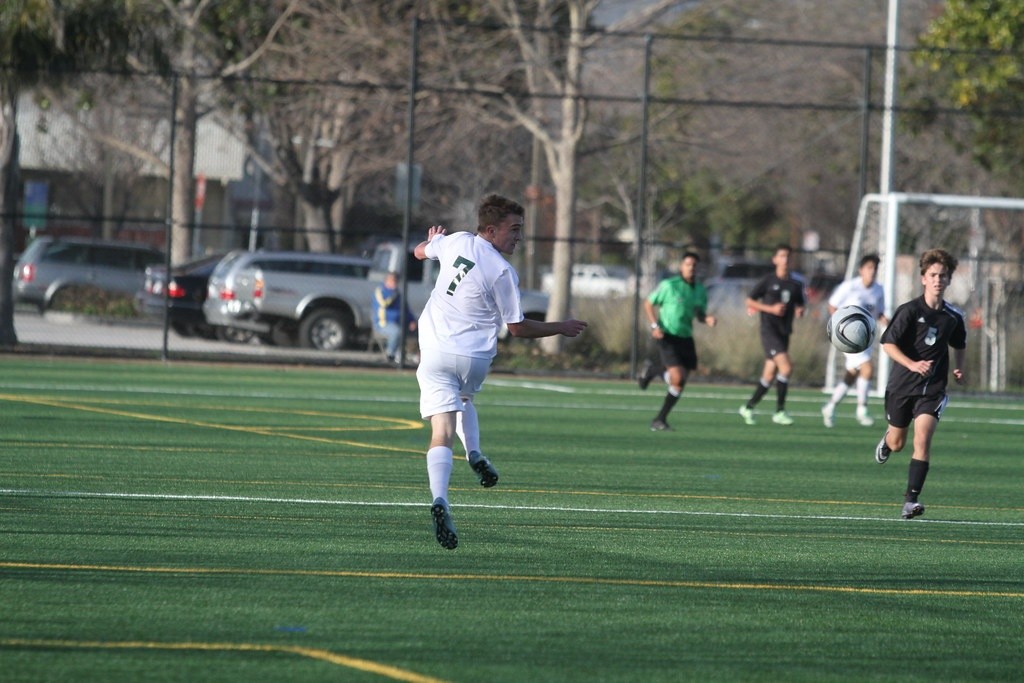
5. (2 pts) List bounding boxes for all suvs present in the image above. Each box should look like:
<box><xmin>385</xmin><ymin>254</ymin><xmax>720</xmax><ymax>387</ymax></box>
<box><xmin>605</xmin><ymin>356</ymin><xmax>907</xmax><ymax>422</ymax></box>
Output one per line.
<box><xmin>13</xmin><ymin>234</ymin><xmax>165</xmax><ymax>314</ymax></box>
<box><xmin>202</xmin><ymin>250</ymin><xmax>373</xmax><ymax>344</ymax></box>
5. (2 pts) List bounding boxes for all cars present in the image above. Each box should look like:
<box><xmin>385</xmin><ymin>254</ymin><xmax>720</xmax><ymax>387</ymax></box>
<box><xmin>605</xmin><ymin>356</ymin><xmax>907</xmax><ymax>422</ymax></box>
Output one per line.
<box><xmin>542</xmin><ymin>263</ymin><xmax>636</xmax><ymax>301</ymax></box>
<box><xmin>699</xmin><ymin>260</ymin><xmax>843</xmax><ymax>317</ymax></box>
<box><xmin>133</xmin><ymin>254</ymin><xmax>228</xmax><ymax>339</ymax></box>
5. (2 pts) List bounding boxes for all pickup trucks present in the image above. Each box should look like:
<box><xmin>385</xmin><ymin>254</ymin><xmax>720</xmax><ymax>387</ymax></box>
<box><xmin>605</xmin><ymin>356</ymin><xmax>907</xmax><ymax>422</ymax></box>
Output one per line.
<box><xmin>255</xmin><ymin>242</ymin><xmax>548</xmax><ymax>351</ymax></box>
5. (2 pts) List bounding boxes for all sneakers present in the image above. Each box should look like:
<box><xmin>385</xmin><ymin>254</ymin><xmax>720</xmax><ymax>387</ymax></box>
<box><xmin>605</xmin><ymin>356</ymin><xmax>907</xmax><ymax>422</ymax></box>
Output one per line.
<box><xmin>856</xmin><ymin>406</ymin><xmax>874</xmax><ymax>425</ymax></box>
<box><xmin>902</xmin><ymin>502</ymin><xmax>925</xmax><ymax>519</ymax></box>
<box><xmin>875</xmin><ymin>429</ymin><xmax>891</xmax><ymax>464</ymax></box>
<box><xmin>431</xmin><ymin>497</ymin><xmax>458</xmax><ymax>550</ymax></box>
<box><xmin>739</xmin><ymin>406</ymin><xmax>756</xmax><ymax>425</ymax></box>
<box><xmin>468</xmin><ymin>451</ymin><xmax>499</xmax><ymax>487</ymax></box>
<box><xmin>772</xmin><ymin>411</ymin><xmax>793</xmax><ymax>424</ymax></box>
<box><xmin>639</xmin><ymin>360</ymin><xmax>652</xmax><ymax>390</ymax></box>
<box><xmin>651</xmin><ymin>421</ymin><xmax>672</xmax><ymax>432</ymax></box>
<box><xmin>821</xmin><ymin>408</ymin><xmax>834</xmax><ymax>428</ymax></box>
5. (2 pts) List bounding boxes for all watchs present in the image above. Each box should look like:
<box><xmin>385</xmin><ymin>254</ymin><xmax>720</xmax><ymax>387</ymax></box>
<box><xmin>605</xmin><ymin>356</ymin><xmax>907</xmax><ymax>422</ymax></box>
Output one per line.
<box><xmin>650</xmin><ymin>322</ymin><xmax>660</xmax><ymax>329</ymax></box>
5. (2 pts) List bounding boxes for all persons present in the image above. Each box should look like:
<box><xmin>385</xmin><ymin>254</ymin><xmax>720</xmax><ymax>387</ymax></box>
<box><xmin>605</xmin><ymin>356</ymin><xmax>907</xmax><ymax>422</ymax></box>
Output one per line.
<box><xmin>645</xmin><ymin>252</ymin><xmax>719</xmax><ymax>430</ymax></box>
<box><xmin>636</xmin><ymin>349</ymin><xmax>661</xmax><ymax>389</ymax></box>
<box><xmin>370</xmin><ymin>272</ymin><xmax>416</xmax><ymax>362</ymax></box>
<box><xmin>740</xmin><ymin>244</ymin><xmax>806</xmax><ymax>423</ymax></box>
<box><xmin>413</xmin><ymin>194</ymin><xmax>588</xmax><ymax>549</ymax></box>
<box><xmin>821</xmin><ymin>255</ymin><xmax>889</xmax><ymax>426</ymax></box>
<box><xmin>876</xmin><ymin>250</ymin><xmax>968</xmax><ymax>520</ymax></box>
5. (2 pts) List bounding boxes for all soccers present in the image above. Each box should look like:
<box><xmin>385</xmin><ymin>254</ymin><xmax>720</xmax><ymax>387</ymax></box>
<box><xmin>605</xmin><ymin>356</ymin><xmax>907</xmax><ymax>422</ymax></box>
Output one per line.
<box><xmin>826</xmin><ymin>305</ymin><xmax>877</xmax><ymax>353</ymax></box>
